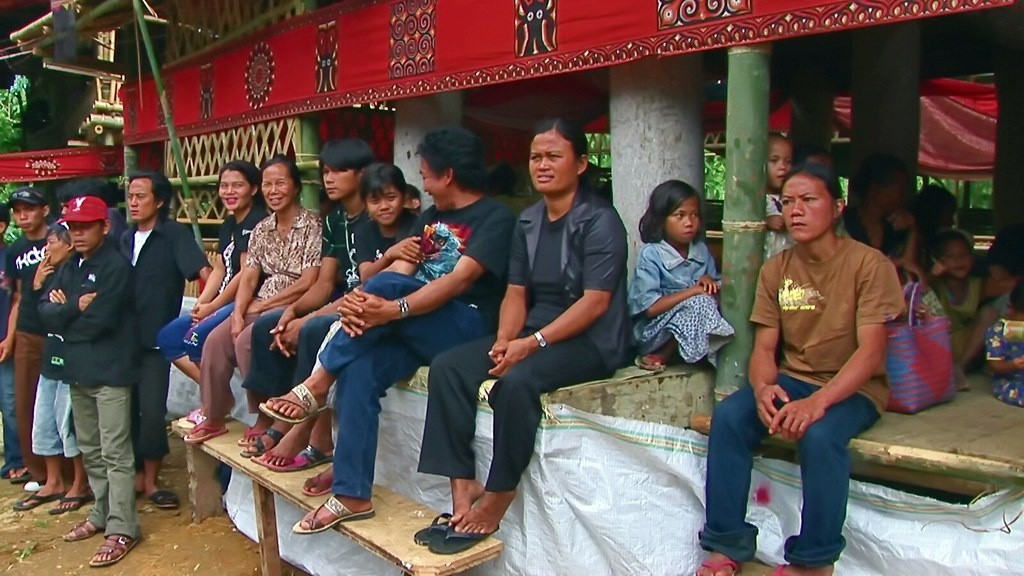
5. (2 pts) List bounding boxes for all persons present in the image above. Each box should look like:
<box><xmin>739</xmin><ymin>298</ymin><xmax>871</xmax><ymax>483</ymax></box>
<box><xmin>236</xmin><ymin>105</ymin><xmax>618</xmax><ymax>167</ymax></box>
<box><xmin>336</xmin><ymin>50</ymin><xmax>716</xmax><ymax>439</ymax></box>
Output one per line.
<box><xmin>257</xmin><ymin>125</ymin><xmax>516</xmax><ymax>534</ymax></box>
<box><xmin>765</xmin><ymin>134</ymin><xmax>1024</xmax><ymax>410</ymax></box>
<box><xmin>0</xmin><ymin>138</ymin><xmax>421</xmax><ymax>567</ymax></box>
<box><xmin>484</xmin><ymin>163</ymin><xmax>539</xmax><ymax>218</ymax></box>
<box><xmin>692</xmin><ymin>163</ymin><xmax>907</xmax><ymax>576</ymax></box>
<box><xmin>626</xmin><ymin>179</ymin><xmax>735</xmax><ymax>371</ymax></box>
<box><xmin>415</xmin><ymin>118</ymin><xmax>629</xmax><ymax>535</ymax></box>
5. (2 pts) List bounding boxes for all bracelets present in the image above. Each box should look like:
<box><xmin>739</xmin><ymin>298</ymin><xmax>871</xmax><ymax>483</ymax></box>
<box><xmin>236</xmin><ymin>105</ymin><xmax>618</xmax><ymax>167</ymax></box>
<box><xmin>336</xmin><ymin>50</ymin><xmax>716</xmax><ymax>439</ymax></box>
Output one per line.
<box><xmin>397</xmin><ymin>296</ymin><xmax>409</xmax><ymax>319</ymax></box>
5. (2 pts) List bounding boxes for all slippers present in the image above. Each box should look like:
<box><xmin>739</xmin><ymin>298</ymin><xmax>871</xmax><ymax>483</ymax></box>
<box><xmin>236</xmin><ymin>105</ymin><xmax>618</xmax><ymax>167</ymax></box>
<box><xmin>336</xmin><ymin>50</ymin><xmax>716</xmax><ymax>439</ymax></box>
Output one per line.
<box><xmin>302</xmin><ymin>473</ymin><xmax>332</xmax><ymax>496</ymax></box>
<box><xmin>250</xmin><ymin>449</ymin><xmax>309</xmax><ymax>471</ymax></box>
<box><xmin>240</xmin><ymin>428</ymin><xmax>285</xmax><ymax>458</ymax></box>
<box><xmin>13</xmin><ymin>491</ymin><xmax>66</xmax><ymax>511</ymax></box>
<box><xmin>48</xmin><ymin>494</ymin><xmax>95</xmax><ymax>515</ymax></box>
<box><xmin>22</xmin><ymin>480</ymin><xmax>47</xmax><ymax>492</ymax></box>
<box><xmin>414</xmin><ymin>513</ymin><xmax>454</xmax><ymax>546</ymax></box>
<box><xmin>8</xmin><ymin>467</ymin><xmax>31</xmax><ymax>485</ymax></box>
<box><xmin>135</xmin><ymin>490</ymin><xmax>145</xmax><ymax>499</ymax></box>
<box><xmin>183</xmin><ymin>424</ymin><xmax>229</xmax><ymax>444</ymax></box>
<box><xmin>149</xmin><ymin>490</ymin><xmax>179</xmax><ymax>508</ymax></box>
<box><xmin>292</xmin><ymin>496</ymin><xmax>375</xmax><ymax>535</ymax></box>
<box><xmin>270</xmin><ymin>445</ymin><xmax>333</xmax><ymax>473</ymax></box>
<box><xmin>773</xmin><ymin>564</ymin><xmax>788</xmax><ymax>576</ymax></box>
<box><xmin>177</xmin><ymin>408</ymin><xmax>231</xmax><ymax>429</ymax></box>
<box><xmin>428</xmin><ymin>524</ymin><xmax>500</xmax><ymax>555</ymax></box>
<box><xmin>238</xmin><ymin>427</ymin><xmax>266</xmax><ymax>447</ymax></box>
<box><xmin>694</xmin><ymin>560</ymin><xmax>742</xmax><ymax>576</ymax></box>
<box><xmin>258</xmin><ymin>383</ymin><xmax>328</xmax><ymax>424</ymax></box>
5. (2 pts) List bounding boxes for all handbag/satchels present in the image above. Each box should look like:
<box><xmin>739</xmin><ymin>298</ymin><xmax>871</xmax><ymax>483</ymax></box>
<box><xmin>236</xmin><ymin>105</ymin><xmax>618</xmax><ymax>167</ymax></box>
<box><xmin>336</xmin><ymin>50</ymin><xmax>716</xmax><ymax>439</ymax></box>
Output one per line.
<box><xmin>884</xmin><ymin>281</ymin><xmax>956</xmax><ymax>414</ymax></box>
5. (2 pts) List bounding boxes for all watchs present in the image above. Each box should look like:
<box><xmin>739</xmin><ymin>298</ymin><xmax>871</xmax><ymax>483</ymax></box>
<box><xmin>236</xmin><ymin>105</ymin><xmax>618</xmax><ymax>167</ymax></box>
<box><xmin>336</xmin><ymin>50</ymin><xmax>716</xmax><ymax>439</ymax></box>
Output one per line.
<box><xmin>533</xmin><ymin>332</ymin><xmax>548</xmax><ymax>349</ymax></box>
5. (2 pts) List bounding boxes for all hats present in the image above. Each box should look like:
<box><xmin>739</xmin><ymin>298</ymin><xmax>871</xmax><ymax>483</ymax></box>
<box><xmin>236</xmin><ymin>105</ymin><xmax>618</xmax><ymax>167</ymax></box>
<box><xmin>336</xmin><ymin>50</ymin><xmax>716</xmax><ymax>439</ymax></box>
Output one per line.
<box><xmin>55</xmin><ymin>196</ymin><xmax>109</xmax><ymax>224</ymax></box>
<box><xmin>7</xmin><ymin>187</ymin><xmax>46</xmax><ymax>209</ymax></box>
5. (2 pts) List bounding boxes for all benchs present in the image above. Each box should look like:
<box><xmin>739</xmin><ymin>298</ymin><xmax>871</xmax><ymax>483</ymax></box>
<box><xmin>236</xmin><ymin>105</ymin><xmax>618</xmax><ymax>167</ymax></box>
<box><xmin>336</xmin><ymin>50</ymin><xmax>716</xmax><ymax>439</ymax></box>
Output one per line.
<box><xmin>171</xmin><ymin>408</ymin><xmax>506</xmax><ymax>576</ymax></box>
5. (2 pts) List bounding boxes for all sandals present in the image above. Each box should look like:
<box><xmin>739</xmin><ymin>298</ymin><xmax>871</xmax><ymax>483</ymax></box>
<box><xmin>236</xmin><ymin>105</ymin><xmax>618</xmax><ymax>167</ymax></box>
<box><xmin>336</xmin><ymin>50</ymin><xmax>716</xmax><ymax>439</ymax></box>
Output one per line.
<box><xmin>88</xmin><ymin>535</ymin><xmax>142</xmax><ymax>567</ymax></box>
<box><xmin>63</xmin><ymin>518</ymin><xmax>100</xmax><ymax>542</ymax></box>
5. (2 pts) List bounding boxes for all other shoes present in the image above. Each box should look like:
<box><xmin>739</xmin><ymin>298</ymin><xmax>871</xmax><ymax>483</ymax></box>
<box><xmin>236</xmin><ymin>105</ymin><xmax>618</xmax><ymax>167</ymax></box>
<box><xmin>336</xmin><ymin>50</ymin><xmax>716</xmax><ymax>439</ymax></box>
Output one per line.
<box><xmin>634</xmin><ymin>355</ymin><xmax>670</xmax><ymax>370</ymax></box>
